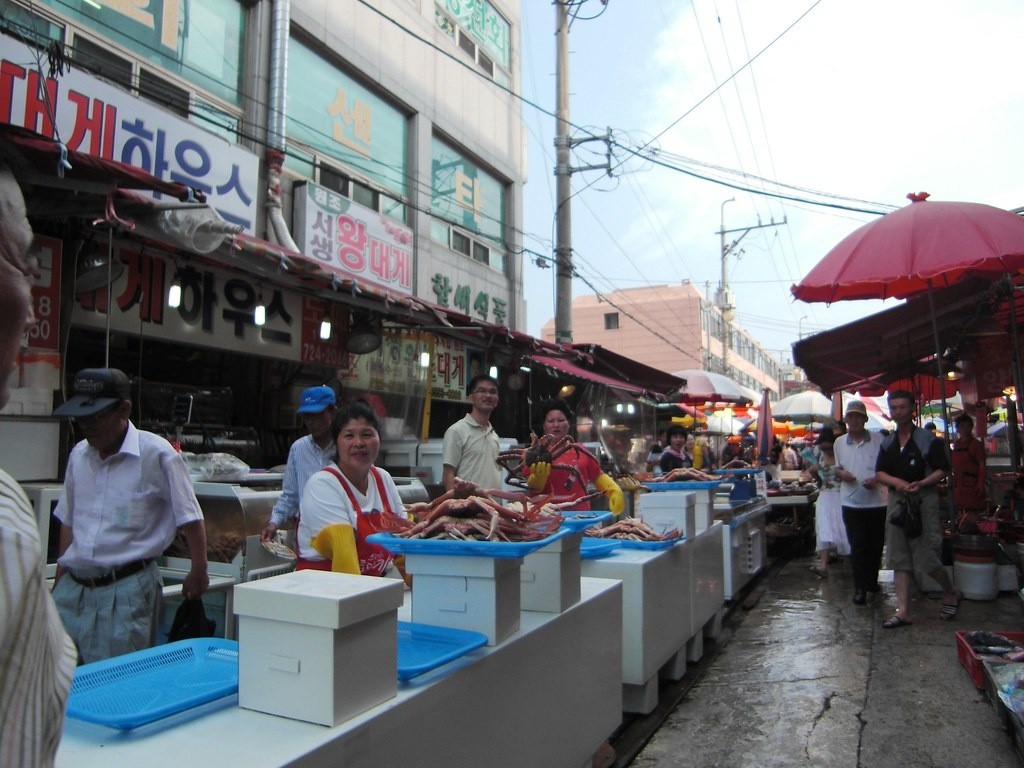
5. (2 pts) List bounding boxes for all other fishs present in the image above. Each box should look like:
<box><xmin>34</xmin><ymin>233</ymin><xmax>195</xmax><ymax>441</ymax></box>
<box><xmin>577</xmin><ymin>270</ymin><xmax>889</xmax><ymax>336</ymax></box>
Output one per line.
<box><xmin>961</xmin><ymin>629</ymin><xmax>1023</xmax><ymax>660</ymax></box>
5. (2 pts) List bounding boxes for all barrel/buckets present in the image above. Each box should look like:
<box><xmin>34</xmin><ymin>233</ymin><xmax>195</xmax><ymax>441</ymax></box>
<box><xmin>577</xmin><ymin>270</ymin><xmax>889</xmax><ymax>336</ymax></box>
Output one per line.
<box><xmin>939</xmin><ymin>515</ymin><xmax>1024</xmax><ymax>601</ymax></box>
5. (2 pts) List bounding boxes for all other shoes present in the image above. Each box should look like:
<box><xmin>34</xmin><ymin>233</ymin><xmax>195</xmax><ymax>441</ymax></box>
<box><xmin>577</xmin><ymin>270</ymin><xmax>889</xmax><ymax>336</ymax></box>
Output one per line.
<box><xmin>854</xmin><ymin>593</ymin><xmax>865</xmax><ymax>605</ymax></box>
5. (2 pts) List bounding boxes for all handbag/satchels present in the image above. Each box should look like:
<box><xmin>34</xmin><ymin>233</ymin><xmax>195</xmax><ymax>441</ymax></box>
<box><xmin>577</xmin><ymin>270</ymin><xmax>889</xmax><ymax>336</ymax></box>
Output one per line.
<box><xmin>888</xmin><ymin>492</ymin><xmax>924</xmax><ymax>539</ymax></box>
<box><xmin>163</xmin><ymin>596</ymin><xmax>216</xmax><ymax>643</ymax></box>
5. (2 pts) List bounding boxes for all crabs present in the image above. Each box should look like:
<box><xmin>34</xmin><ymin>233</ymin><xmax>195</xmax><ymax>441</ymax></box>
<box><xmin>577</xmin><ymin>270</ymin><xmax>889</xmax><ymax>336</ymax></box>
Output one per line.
<box><xmin>632</xmin><ymin>466</ymin><xmax>732</xmax><ymax>483</ymax></box>
<box><xmin>582</xmin><ymin>515</ymin><xmax>684</xmax><ymax>541</ymax></box>
<box><xmin>393</xmin><ymin>477</ymin><xmax>608</xmax><ymax>545</ymax></box>
<box><xmin>496</xmin><ymin>432</ymin><xmax>601</xmax><ymax>496</ymax></box>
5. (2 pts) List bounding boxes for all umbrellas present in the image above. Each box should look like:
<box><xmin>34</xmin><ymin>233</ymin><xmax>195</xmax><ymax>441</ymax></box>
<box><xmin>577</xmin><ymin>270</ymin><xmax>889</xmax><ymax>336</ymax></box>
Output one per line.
<box><xmin>668</xmin><ymin>369</ymin><xmax>834</xmax><ymax>446</ymax></box>
<box><xmin>752</xmin><ymin>387</ymin><xmax>773</xmax><ymax>466</ymax></box>
<box><xmin>791</xmin><ymin>192</ymin><xmax>1024</xmax><ymax>557</ymax></box>
<box><xmin>844</xmin><ymin>355</ymin><xmax>958</xmax><ymax>428</ymax></box>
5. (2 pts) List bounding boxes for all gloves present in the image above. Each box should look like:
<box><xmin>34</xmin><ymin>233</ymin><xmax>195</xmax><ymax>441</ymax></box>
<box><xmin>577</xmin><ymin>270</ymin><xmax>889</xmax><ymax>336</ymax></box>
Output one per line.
<box><xmin>594</xmin><ymin>473</ymin><xmax>625</xmax><ymax>516</ymax></box>
<box><xmin>527</xmin><ymin>462</ymin><xmax>550</xmax><ymax>492</ymax></box>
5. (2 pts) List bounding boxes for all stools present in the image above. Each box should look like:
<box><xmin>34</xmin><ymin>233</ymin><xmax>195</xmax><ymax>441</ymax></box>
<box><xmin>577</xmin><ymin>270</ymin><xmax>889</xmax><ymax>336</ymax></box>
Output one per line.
<box><xmin>19</xmin><ymin>483</ymin><xmax>64</xmax><ymax>579</ymax></box>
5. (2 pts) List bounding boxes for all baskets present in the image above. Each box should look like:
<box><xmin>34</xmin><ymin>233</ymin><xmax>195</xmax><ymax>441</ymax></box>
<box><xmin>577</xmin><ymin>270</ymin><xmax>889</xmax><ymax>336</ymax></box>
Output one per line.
<box><xmin>366</xmin><ymin>510</ymin><xmax>688</xmax><ymax>558</ymax></box>
<box><xmin>712</xmin><ymin>469</ymin><xmax>762</xmax><ymax>475</ymax></box>
<box><xmin>65</xmin><ymin>638</ymin><xmax>239</xmax><ymax>730</ymax></box>
<box><xmin>396</xmin><ymin>620</ymin><xmax>488</xmax><ymax>680</ymax></box>
<box><xmin>641</xmin><ymin>480</ymin><xmax>722</xmax><ymax>491</ymax></box>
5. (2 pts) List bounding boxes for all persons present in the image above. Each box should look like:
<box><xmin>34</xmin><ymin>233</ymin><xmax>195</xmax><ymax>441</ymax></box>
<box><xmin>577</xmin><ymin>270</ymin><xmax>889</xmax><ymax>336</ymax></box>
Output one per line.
<box><xmin>809</xmin><ymin>400</ymin><xmax>988</xmax><ymax>604</ymax></box>
<box><xmin>442</xmin><ymin>375</ymin><xmax>508</xmax><ymax>506</ymax></box>
<box><xmin>876</xmin><ymin>389</ymin><xmax>963</xmax><ymax>628</ymax></box>
<box><xmin>0</xmin><ymin>157</ymin><xmax>78</xmax><ymax>768</ymax></box>
<box><xmin>1013</xmin><ymin>477</ymin><xmax>1024</xmax><ymax>500</ymax></box>
<box><xmin>521</xmin><ymin>397</ymin><xmax>625</xmax><ymax>516</ymax></box>
<box><xmin>261</xmin><ymin>385</ymin><xmax>412</xmax><ymax>588</ymax></box>
<box><xmin>646</xmin><ymin>425</ymin><xmax>823</xmax><ymax>496</ymax></box>
<box><xmin>49</xmin><ymin>368</ymin><xmax>211</xmax><ymax>667</ymax></box>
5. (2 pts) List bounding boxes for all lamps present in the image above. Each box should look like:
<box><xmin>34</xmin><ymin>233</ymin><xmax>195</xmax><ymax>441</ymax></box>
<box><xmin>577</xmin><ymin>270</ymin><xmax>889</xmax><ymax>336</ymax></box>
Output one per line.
<box><xmin>343</xmin><ymin>308</ymin><xmax>384</xmax><ymax>354</ymax></box>
<box><xmin>941</xmin><ymin>362</ymin><xmax>966</xmax><ymax>381</ymax></box>
<box><xmin>552</xmin><ymin>375</ymin><xmax>577</xmax><ymax>400</ymax></box>
<box><xmin>75</xmin><ymin>227</ymin><xmax>125</xmax><ymax>293</ymax></box>
<box><xmin>963</xmin><ymin>287</ymin><xmax>1011</xmax><ymax>336</ymax></box>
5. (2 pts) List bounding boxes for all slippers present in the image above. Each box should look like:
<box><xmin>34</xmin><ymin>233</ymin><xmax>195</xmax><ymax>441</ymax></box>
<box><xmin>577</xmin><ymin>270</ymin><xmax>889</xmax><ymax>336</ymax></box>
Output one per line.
<box><xmin>882</xmin><ymin>616</ymin><xmax>912</xmax><ymax>628</ymax></box>
<box><xmin>938</xmin><ymin>591</ymin><xmax>964</xmax><ymax>621</ymax></box>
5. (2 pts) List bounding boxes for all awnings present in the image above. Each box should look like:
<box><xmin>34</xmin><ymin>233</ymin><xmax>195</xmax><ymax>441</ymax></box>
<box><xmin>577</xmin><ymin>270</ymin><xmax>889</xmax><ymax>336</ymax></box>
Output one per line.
<box><xmin>0</xmin><ymin>124</ymin><xmax>708</xmax><ymax>419</ymax></box>
<box><xmin>792</xmin><ymin>269</ymin><xmax>1024</xmax><ymax>447</ymax></box>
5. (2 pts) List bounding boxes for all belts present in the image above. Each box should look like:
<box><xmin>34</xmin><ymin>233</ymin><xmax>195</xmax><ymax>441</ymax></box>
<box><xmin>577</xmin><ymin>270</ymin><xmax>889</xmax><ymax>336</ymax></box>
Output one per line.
<box><xmin>68</xmin><ymin>556</ymin><xmax>154</xmax><ymax>587</ymax></box>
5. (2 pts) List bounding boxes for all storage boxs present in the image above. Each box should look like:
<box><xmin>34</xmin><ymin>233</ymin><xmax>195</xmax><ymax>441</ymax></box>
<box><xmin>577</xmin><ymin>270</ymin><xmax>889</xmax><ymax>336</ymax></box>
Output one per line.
<box><xmin>634</xmin><ymin>489</ymin><xmax>716</xmax><ymax>540</ymax></box>
<box><xmin>954</xmin><ymin>630</ymin><xmax>1024</xmax><ymax>764</ymax></box>
<box><xmin>233</xmin><ymin>569</ymin><xmax>405</xmax><ymax>730</ymax></box>
<box><xmin>0</xmin><ymin>347</ymin><xmax>60</xmax><ymax>416</ymax></box>
<box><xmin>520</xmin><ymin>532</ymin><xmax>582</xmax><ymax>611</ymax></box>
<box><xmin>404</xmin><ymin>553</ymin><xmax>525</xmax><ymax>643</ymax></box>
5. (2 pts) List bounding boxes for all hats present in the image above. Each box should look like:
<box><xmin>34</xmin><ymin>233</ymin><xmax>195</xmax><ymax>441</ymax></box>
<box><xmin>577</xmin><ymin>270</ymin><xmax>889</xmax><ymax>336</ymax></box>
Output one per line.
<box><xmin>51</xmin><ymin>369</ymin><xmax>131</xmax><ymax>418</ymax></box>
<box><xmin>846</xmin><ymin>401</ymin><xmax>866</xmax><ymax>415</ymax></box>
<box><xmin>296</xmin><ymin>384</ymin><xmax>335</xmax><ymax>414</ymax></box>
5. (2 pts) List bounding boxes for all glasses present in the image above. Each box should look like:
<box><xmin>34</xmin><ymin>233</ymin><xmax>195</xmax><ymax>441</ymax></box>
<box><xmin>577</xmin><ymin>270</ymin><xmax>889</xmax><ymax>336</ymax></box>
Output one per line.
<box><xmin>471</xmin><ymin>388</ymin><xmax>498</xmax><ymax>395</ymax></box>
